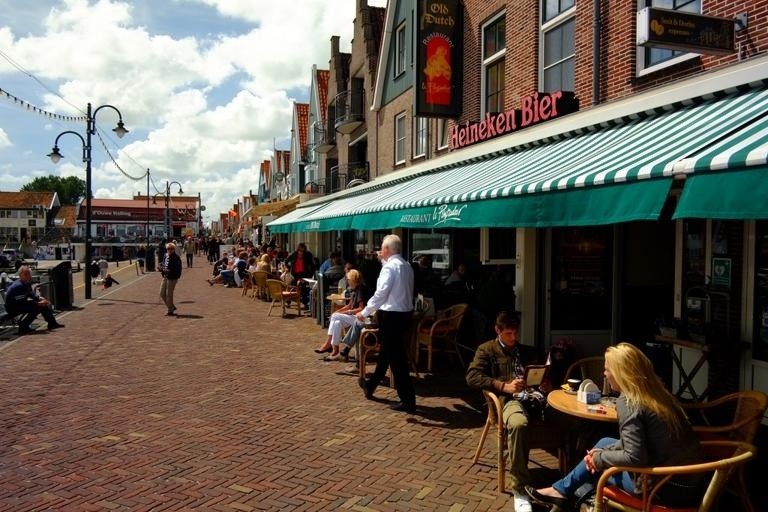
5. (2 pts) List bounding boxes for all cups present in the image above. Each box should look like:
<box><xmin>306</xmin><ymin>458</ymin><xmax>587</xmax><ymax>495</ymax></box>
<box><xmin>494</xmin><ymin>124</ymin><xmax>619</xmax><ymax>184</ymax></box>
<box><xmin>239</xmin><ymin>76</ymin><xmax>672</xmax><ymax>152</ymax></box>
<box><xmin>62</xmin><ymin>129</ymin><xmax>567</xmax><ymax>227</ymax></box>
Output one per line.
<box><xmin>566</xmin><ymin>379</ymin><xmax>580</xmax><ymax>390</ymax></box>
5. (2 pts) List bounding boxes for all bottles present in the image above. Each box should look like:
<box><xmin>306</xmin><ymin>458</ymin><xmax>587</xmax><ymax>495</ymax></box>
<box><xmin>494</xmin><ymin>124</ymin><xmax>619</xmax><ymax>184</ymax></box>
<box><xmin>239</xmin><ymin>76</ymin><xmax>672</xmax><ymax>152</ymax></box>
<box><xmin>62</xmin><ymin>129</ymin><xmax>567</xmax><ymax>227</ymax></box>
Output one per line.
<box><xmin>602</xmin><ymin>377</ymin><xmax>610</xmax><ymax>395</ymax></box>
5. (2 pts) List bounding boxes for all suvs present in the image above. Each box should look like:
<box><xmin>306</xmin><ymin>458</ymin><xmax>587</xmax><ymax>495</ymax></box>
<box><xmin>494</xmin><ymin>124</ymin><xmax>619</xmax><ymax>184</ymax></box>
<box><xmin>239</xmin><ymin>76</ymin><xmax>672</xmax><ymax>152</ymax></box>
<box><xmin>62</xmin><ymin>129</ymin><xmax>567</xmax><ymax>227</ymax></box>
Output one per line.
<box><xmin>2</xmin><ymin>249</ymin><xmax>25</xmax><ymax>264</ymax></box>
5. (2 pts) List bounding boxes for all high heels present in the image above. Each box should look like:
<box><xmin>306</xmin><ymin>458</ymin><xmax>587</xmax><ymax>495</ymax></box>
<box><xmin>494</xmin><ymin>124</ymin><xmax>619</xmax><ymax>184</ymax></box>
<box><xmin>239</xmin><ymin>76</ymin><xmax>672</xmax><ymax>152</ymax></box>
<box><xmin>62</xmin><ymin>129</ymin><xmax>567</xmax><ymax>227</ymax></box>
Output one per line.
<box><xmin>524</xmin><ymin>484</ymin><xmax>572</xmax><ymax>512</ymax></box>
<box><xmin>315</xmin><ymin>346</ymin><xmax>332</xmax><ymax>353</ymax></box>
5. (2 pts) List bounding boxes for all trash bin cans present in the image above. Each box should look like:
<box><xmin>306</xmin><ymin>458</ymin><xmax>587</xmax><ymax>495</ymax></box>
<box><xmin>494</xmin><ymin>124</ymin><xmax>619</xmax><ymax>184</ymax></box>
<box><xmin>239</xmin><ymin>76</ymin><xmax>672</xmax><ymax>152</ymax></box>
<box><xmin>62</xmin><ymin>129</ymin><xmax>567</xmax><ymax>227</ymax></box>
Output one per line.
<box><xmin>146</xmin><ymin>248</ymin><xmax>157</xmax><ymax>271</ymax></box>
<box><xmin>48</xmin><ymin>261</ymin><xmax>74</xmax><ymax>311</ymax></box>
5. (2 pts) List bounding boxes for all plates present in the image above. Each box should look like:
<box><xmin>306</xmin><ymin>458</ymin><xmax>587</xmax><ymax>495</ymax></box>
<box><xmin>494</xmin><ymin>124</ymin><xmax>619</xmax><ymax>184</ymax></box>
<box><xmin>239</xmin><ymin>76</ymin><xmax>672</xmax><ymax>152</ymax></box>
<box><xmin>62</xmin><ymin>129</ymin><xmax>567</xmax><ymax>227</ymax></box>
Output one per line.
<box><xmin>563</xmin><ymin>389</ymin><xmax>577</xmax><ymax>395</ymax></box>
<box><xmin>577</xmin><ymin>379</ymin><xmax>599</xmax><ymax>403</ymax></box>
<box><xmin>599</xmin><ymin>397</ymin><xmax>618</xmax><ymax>408</ymax></box>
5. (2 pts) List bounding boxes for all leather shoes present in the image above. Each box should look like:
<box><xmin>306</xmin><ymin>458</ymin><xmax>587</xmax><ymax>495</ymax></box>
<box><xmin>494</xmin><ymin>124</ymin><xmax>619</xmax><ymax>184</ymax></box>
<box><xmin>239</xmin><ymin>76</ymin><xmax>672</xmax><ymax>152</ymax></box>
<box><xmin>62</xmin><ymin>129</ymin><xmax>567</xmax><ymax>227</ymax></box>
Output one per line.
<box><xmin>391</xmin><ymin>404</ymin><xmax>417</xmax><ymax>414</ymax></box>
<box><xmin>359</xmin><ymin>377</ymin><xmax>372</xmax><ymax>399</ymax></box>
<box><xmin>323</xmin><ymin>355</ymin><xmax>332</xmax><ymax>362</ymax></box>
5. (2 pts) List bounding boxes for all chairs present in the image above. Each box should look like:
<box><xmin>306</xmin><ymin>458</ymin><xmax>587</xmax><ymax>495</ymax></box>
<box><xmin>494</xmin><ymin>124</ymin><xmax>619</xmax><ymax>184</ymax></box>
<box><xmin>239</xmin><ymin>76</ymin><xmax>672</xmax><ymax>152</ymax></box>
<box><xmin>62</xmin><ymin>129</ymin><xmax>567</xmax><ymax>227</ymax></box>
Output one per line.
<box><xmin>0</xmin><ymin>289</ymin><xmax>26</xmax><ymax>327</ymax></box>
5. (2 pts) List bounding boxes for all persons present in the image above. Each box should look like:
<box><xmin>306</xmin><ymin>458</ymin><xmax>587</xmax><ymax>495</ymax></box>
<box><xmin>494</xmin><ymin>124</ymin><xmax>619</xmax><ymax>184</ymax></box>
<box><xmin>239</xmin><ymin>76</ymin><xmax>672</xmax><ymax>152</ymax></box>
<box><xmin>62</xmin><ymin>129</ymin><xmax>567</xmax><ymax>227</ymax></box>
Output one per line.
<box><xmin>101</xmin><ymin>274</ymin><xmax>120</xmax><ymax>289</ymax></box>
<box><xmin>154</xmin><ymin>230</ymin><xmax>364</xmax><ymax>317</ymax></box>
<box><xmin>91</xmin><ymin>259</ymin><xmax>100</xmax><ymax>285</ymax></box>
<box><xmin>355</xmin><ymin>232</ymin><xmax>419</xmax><ymax>415</ymax></box>
<box><xmin>327</xmin><ymin>314</ymin><xmax>378</xmax><ymax>373</ymax></box>
<box><xmin>135</xmin><ymin>245</ymin><xmax>149</xmax><ymax>274</ymax></box>
<box><xmin>3</xmin><ymin>264</ymin><xmax>66</xmax><ymax>335</ymax></box>
<box><xmin>522</xmin><ymin>340</ymin><xmax>706</xmax><ymax>512</ymax></box>
<box><xmin>463</xmin><ymin>310</ymin><xmax>577</xmax><ymax>512</ymax></box>
<box><xmin>95</xmin><ymin>255</ymin><xmax>109</xmax><ymax>280</ymax></box>
<box><xmin>313</xmin><ymin>268</ymin><xmax>371</xmax><ymax>360</ymax></box>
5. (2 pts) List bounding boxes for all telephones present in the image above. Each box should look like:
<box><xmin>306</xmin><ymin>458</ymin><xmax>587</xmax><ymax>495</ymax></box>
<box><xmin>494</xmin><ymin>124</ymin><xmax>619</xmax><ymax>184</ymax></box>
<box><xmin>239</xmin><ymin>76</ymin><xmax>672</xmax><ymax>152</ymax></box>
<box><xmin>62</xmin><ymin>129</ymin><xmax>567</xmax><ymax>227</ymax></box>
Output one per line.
<box><xmin>319</xmin><ymin>272</ymin><xmax>347</xmax><ymax>329</ymax></box>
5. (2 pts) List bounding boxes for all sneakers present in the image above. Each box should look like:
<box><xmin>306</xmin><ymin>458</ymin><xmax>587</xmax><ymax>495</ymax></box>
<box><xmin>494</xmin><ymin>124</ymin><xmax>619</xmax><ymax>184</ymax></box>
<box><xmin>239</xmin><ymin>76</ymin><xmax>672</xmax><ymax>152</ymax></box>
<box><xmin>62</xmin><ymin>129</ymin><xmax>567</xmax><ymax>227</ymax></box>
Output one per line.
<box><xmin>327</xmin><ymin>351</ymin><xmax>349</xmax><ymax>361</ymax></box>
<box><xmin>514</xmin><ymin>493</ymin><xmax>532</xmax><ymax>512</ymax></box>
<box><xmin>18</xmin><ymin>327</ymin><xmax>36</xmax><ymax>334</ymax></box>
<box><xmin>346</xmin><ymin>366</ymin><xmax>360</xmax><ymax>373</ymax></box>
<box><xmin>48</xmin><ymin>324</ymin><xmax>65</xmax><ymax>329</ymax></box>
<box><xmin>164</xmin><ymin>307</ymin><xmax>177</xmax><ymax>316</ymax></box>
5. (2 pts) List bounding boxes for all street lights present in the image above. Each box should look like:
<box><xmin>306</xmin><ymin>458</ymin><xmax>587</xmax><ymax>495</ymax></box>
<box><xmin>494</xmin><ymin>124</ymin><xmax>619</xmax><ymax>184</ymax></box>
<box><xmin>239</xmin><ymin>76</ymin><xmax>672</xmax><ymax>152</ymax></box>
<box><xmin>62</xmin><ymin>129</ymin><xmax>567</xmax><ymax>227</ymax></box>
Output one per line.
<box><xmin>152</xmin><ymin>179</ymin><xmax>185</xmax><ymax>243</ymax></box>
<box><xmin>48</xmin><ymin>103</ymin><xmax>130</xmax><ymax>300</ymax></box>
